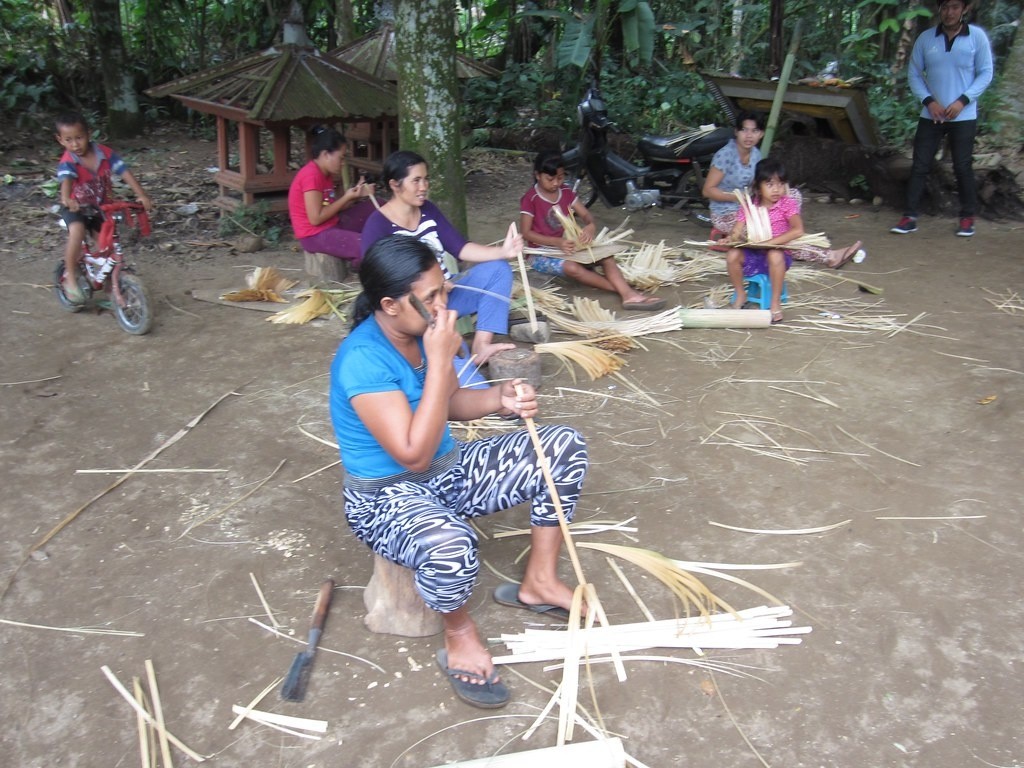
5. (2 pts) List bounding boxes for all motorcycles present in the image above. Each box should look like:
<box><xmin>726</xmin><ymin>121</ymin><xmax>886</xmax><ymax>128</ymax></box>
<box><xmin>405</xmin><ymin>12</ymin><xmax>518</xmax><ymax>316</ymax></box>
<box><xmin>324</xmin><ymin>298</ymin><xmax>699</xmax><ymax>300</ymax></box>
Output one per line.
<box><xmin>533</xmin><ymin>79</ymin><xmax>737</xmax><ymax>228</ymax></box>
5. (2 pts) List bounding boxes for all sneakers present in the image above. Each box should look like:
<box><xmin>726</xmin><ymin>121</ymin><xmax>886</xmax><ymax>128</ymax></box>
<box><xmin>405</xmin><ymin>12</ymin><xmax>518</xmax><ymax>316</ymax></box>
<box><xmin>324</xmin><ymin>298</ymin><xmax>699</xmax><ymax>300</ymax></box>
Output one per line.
<box><xmin>955</xmin><ymin>217</ymin><xmax>975</xmax><ymax>236</ymax></box>
<box><xmin>889</xmin><ymin>217</ymin><xmax>919</xmax><ymax>233</ymax></box>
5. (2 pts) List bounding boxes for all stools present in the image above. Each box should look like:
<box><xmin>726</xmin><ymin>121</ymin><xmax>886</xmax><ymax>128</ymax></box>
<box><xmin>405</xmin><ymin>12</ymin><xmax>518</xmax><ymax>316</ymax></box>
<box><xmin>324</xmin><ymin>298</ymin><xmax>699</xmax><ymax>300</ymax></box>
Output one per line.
<box><xmin>730</xmin><ymin>272</ymin><xmax>787</xmax><ymax>310</ymax></box>
<box><xmin>708</xmin><ymin>227</ymin><xmax>735</xmax><ymax>253</ymax></box>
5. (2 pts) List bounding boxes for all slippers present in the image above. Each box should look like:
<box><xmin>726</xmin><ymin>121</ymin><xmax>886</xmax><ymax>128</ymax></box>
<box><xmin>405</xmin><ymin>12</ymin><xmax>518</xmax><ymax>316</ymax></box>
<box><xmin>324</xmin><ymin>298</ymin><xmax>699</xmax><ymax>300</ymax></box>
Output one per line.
<box><xmin>436</xmin><ymin>649</ymin><xmax>511</xmax><ymax>708</ymax></box>
<box><xmin>771</xmin><ymin>310</ymin><xmax>783</xmax><ymax>324</ymax></box>
<box><xmin>729</xmin><ymin>301</ymin><xmax>751</xmax><ymax>309</ymax></box>
<box><xmin>623</xmin><ymin>296</ymin><xmax>664</xmax><ymax>310</ymax></box>
<box><xmin>831</xmin><ymin>241</ymin><xmax>862</xmax><ymax>269</ymax></box>
<box><xmin>493</xmin><ymin>582</ymin><xmax>584</xmax><ymax>622</ymax></box>
<box><xmin>62</xmin><ymin>279</ymin><xmax>85</xmax><ymax>305</ymax></box>
<box><xmin>93</xmin><ymin>297</ymin><xmax>132</xmax><ymax>311</ymax></box>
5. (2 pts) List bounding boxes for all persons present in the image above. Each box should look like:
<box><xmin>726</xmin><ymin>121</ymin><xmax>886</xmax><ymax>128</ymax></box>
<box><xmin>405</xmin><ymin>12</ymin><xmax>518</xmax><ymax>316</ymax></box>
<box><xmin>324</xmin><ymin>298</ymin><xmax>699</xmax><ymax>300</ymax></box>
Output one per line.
<box><xmin>54</xmin><ymin>110</ymin><xmax>155</xmax><ymax>304</ymax></box>
<box><xmin>520</xmin><ymin>150</ymin><xmax>667</xmax><ymax>310</ymax></box>
<box><xmin>702</xmin><ymin>110</ymin><xmax>862</xmax><ymax>268</ymax></box>
<box><xmin>890</xmin><ymin>1</ymin><xmax>994</xmax><ymax>236</ymax></box>
<box><xmin>287</xmin><ymin>123</ymin><xmax>387</xmax><ymax>272</ymax></box>
<box><xmin>359</xmin><ymin>150</ymin><xmax>525</xmax><ymax>365</ymax></box>
<box><xmin>330</xmin><ymin>234</ymin><xmax>599</xmax><ymax>708</ymax></box>
<box><xmin>718</xmin><ymin>156</ymin><xmax>804</xmax><ymax>325</ymax></box>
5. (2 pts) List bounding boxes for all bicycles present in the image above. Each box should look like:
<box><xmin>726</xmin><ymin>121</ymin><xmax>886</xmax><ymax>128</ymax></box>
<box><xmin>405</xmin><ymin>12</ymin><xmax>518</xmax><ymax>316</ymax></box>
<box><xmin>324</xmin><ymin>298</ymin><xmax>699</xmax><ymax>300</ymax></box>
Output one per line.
<box><xmin>51</xmin><ymin>201</ymin><xmax>154</xmax><ymax>336</ymax></box>
